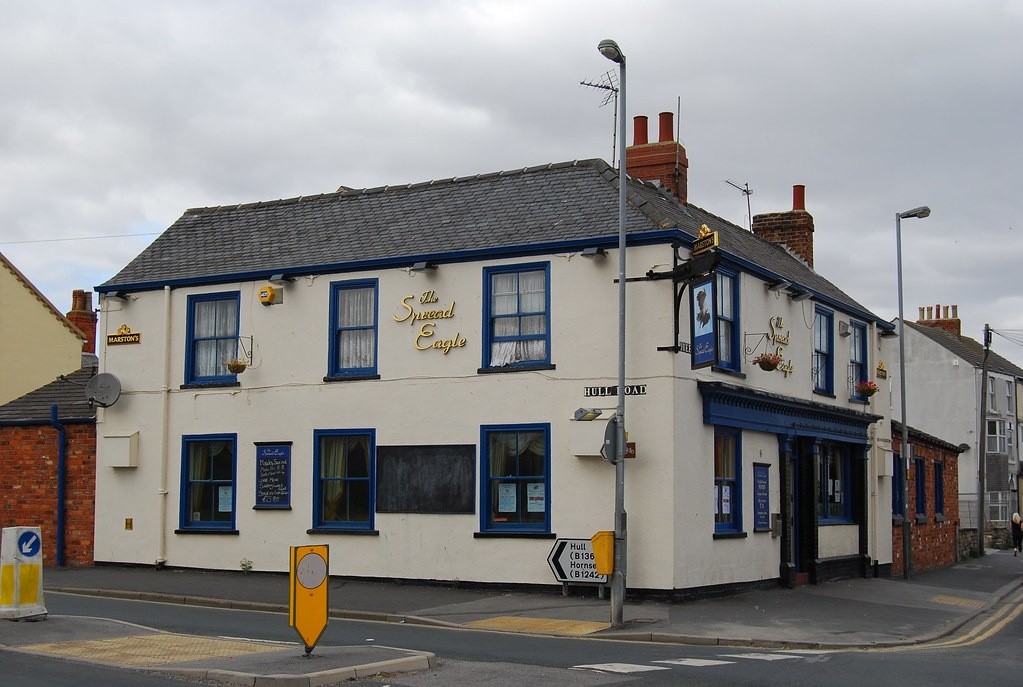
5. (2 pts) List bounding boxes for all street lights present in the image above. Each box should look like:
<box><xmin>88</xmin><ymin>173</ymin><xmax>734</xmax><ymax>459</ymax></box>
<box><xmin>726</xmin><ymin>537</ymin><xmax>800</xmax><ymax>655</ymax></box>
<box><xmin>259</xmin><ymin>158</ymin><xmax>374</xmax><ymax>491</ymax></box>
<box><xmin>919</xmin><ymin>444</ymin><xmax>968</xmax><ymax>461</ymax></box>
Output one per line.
<box><xmin>896</xmin><ymin>206</ymin><xmax>931</xmax><ymax>579</ymax></box>
<box><xmin>597</xmin><ymin>39</ymin><xmax>627</xmax><ymax>628</ymax></box>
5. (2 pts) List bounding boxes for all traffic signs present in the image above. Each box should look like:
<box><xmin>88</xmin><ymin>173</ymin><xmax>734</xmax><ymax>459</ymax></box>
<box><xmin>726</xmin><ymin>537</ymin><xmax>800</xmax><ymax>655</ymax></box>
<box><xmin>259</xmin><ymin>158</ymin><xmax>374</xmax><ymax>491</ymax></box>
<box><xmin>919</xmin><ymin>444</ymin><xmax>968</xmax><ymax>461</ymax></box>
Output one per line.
<box><xmin>547</xmin><ymin>538</ymin><xmax>608</xmax><ymax>583</ymax></box>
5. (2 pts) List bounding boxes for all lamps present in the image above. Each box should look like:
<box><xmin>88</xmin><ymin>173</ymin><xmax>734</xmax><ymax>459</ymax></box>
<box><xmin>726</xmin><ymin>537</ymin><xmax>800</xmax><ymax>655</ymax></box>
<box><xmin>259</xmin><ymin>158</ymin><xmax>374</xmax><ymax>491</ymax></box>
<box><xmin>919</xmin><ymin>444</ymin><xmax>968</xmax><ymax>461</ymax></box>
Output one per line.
<box><xmin>787</xmin><ymin>288</ymin><xmax>812</xmax><ymax>301</ymax></box>
<box><xmin>268</xmin><ymin>275</ymin><xmax>297</xmax><ymax>287</ymax></box>
<box><xmin>105</xmin><ymin>292</ymin><xmax>129</xmax><ymax>303</ymax></box>
<box><xmin>580</xmin><ymin>248</ymin><xmax>608</xmax><ymax>260</ymax></box>
<box><xmin>410</xmin><ymin>262</ymin><xmax>437</xmax><ymax>273</ymax></box>
<box><xmin>765</xmin><ymin>278</ymin><xmax>791</xmax><ymax>293</ymax></box>
<box><xmin>877</xmin><ymin>330</ymin><xmax>898</xmax><ymax>340</ymax></box>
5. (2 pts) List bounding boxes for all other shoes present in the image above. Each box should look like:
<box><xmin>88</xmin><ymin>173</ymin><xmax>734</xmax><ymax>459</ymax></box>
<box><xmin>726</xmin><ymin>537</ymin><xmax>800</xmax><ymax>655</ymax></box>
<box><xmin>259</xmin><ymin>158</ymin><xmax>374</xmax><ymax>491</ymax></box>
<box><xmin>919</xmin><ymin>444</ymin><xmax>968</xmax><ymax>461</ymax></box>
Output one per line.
<box><xmin>1014</xmin><ymin>548</ymin><xmax>1017</xmax><ymax>556</ymax></box>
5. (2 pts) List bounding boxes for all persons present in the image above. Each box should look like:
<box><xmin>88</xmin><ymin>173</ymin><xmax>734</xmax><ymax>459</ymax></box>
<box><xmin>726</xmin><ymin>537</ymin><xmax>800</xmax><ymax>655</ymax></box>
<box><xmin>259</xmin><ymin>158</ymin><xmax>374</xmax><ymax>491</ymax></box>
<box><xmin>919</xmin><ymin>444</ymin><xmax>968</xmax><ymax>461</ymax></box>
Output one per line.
<box><xmin>1009</xmin><ymin>512</ymin><xmax>1023</xmax><ymax>556</ymax></box>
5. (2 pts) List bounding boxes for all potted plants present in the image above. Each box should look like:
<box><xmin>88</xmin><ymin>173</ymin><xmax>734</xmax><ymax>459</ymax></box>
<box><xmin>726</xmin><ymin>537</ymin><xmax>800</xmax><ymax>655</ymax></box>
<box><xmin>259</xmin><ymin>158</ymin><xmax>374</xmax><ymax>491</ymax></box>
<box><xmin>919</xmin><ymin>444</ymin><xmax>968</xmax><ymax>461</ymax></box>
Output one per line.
<box><xmin>225</xmin><ymin>359</ymin><xmax>247</xmax><ymax>373</ymax></box>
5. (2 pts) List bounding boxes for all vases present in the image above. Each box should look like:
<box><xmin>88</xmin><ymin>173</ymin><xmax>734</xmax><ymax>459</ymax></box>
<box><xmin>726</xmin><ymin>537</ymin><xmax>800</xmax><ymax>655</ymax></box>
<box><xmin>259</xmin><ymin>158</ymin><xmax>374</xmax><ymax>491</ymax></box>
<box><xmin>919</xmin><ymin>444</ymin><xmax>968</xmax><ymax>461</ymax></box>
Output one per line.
<box><xmin>760</xmin><ymin>364</ymin><xmax>777</xmax><ymax>372</ymax></box>
<box><xmin>860</xmin><ymin>391</ymin><xmax>876</xmax><ymax>397</ymax></box>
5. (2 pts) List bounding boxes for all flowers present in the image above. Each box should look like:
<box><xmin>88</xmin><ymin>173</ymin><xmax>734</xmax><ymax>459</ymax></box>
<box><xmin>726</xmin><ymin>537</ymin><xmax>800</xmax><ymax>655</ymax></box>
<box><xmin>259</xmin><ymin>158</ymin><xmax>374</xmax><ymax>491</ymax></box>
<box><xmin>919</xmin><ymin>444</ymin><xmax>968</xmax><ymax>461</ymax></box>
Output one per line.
<box><xmin>750</xmin><ymin>352</ymin><xmax>779</xmax><ymax>365</ymax></box>
<box><xmin>854</xmin><ymin>379</ymin><xmax>880</xmax><ymax>391</ymax></box>
